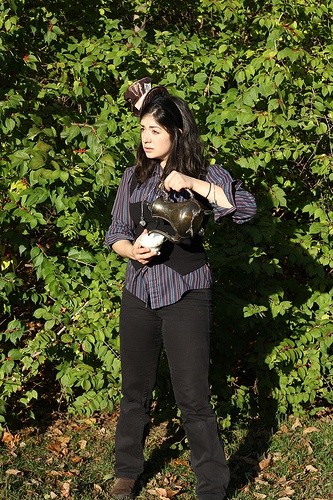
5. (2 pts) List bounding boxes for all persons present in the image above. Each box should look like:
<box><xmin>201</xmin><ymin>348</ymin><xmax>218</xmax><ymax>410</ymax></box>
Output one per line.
<box><xmin>104</xmin><ymin>94</ymin><xmax>254</xmax><ymax>500</ymax></box>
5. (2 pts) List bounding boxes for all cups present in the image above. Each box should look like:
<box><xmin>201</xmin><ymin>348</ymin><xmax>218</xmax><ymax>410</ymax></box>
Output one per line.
<box><xmin>139</xmin><ymin>231</ymin><xmax>164</xmax><ymax>255</ymax></box>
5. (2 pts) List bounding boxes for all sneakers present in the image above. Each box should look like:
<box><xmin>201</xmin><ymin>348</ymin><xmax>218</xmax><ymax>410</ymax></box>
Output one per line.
<box><xmin>109</xmin><ymin>477</ymin><xmax>137</xmax><ymax>498</ymax></box>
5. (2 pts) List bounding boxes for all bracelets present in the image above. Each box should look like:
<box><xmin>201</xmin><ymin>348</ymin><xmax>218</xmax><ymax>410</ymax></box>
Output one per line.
<box><xmin>202</xmin><ymin>181</ymin><xmax>211</xmax><ymax>201</ymax></box>
<box><xmin>211</xmin><ymin>182</ymin><xmax>219</xmax><ymax>205</ymax></box>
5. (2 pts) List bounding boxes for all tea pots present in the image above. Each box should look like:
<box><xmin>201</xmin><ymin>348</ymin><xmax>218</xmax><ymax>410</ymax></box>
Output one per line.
<box><xmin>146</xmin><ymin>182</ymin><xmax>204</xmax><ymax>245</ymax></box>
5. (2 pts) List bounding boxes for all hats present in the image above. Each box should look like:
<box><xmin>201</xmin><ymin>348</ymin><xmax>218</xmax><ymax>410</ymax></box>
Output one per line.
<box><xmin>123</xmin><ymin>79</ymin><xmax>169</xmax><ymax>120</ymax></box>
